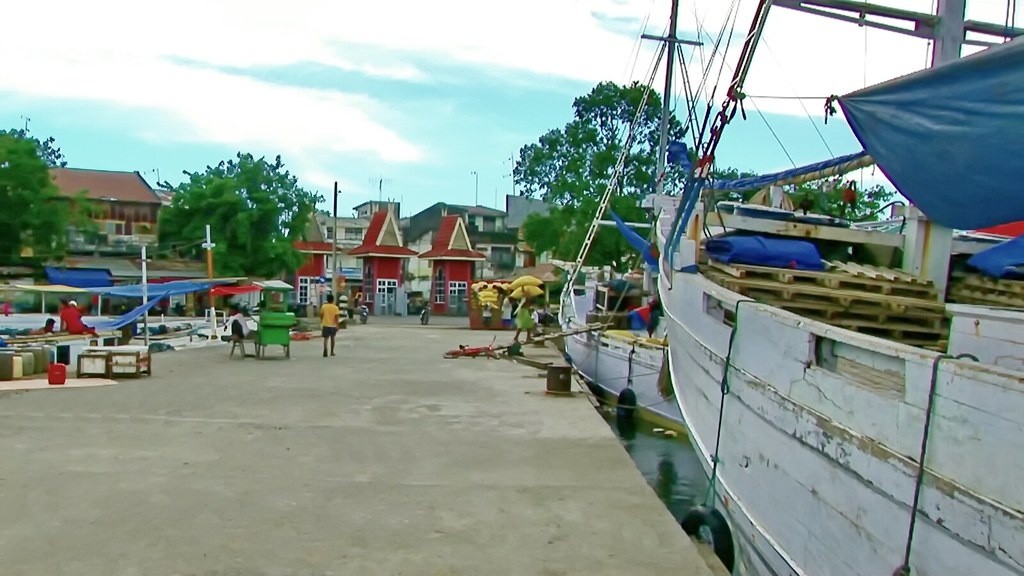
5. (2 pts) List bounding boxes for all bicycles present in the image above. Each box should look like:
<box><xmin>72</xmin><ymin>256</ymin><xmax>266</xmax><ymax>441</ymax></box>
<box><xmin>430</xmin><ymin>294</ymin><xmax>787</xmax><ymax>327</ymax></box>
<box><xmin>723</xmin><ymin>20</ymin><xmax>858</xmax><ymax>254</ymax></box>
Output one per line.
<box><xmin>419</xmin><ymin>306</ymin><xmax>431</xmax><ymax>325</ymax></box>
<box><xmin>442</xmin><ymin>335</ymin><xmax>500</xmax><ymax>360</ymax></box>
<box><xmin>360</xmin><ymin>306</ymin><xmax>367</xmax><ymax>324</ymax></box>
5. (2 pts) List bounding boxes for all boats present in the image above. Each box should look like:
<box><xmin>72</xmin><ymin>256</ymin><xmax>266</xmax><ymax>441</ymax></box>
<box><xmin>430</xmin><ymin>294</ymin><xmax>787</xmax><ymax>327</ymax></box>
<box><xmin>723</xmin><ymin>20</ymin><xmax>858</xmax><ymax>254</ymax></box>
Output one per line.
<box><xmin>196</xmin><ymin>309</ymin><xmax>258</xmax><ymax>338</ymax></box>
<box><xmin>659</xmin><ymin>0</ymin><xmax>1024</xmax><ymax>576</ymax></box>
<box><xmin>129</xmin><ymin>320</ymin><xmax>198</xmax><ymax>348</ymax></box>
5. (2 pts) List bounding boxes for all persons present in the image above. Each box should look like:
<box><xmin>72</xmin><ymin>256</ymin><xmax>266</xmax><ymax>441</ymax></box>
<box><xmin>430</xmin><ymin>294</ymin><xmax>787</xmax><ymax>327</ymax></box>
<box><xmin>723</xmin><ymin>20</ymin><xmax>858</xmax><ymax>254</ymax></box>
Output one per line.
<box><xmin>474</xmin><ymin>285</ymin><xmax>538</xmax><ymax>344</ymax></box>
<box><xmin>320</xmin><ymin>295</ymin><xmax>339</xmax><ymax>357</ymax></box>
<box><xmin>0</xmin><ymin>317</ymin><xmax>56</xmax><ymax>335</ymax></box>
<box><xmin>59</xmin><ymin>296</ymin><xmax>99</xmax><ymax>338</ymax></box>
<box><xmin>221</xmin><ymin>305</ymin><xmax>249</xmax><ymax>343</ymax></box>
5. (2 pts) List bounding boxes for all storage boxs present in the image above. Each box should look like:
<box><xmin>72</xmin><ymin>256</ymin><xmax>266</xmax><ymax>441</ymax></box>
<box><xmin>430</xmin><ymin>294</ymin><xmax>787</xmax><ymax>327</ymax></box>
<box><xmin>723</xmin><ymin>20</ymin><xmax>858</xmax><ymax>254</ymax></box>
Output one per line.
<box><xmin>80</xmin><ymin>346</ymin><xmax>149</xmax><ymax>373</ymax></box>
<box><xmin>655</xmin><ymin>317</ymin><xmax>667</xmax><ymax>338</ymax></box>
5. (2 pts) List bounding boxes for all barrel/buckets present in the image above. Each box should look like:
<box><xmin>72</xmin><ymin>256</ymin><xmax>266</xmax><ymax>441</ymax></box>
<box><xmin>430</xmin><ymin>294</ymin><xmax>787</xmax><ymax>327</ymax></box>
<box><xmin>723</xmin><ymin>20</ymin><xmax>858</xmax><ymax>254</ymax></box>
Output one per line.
<box><xmin>47</xmin><ymin>363</ymin><xmax>66</xmax><ymax>384</ymax></box>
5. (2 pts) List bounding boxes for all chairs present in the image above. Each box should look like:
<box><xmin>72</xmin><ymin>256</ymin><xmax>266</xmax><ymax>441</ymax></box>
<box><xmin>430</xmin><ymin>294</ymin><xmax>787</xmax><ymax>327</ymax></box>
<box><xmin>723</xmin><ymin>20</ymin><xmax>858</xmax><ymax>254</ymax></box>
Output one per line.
<box><xmin>231</xmin><ymin>320</ymin><xmax>257</xmax><ymax>356</ymax></box>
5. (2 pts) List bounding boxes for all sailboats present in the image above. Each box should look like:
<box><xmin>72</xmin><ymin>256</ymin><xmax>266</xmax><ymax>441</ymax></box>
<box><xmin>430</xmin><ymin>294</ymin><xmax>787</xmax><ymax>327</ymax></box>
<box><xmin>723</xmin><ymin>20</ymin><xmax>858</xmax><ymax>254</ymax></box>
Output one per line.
<box><xmin>558</xmin><ymin>0</ymin><xmax>737</xmax><ymax>438</ymax></box>
